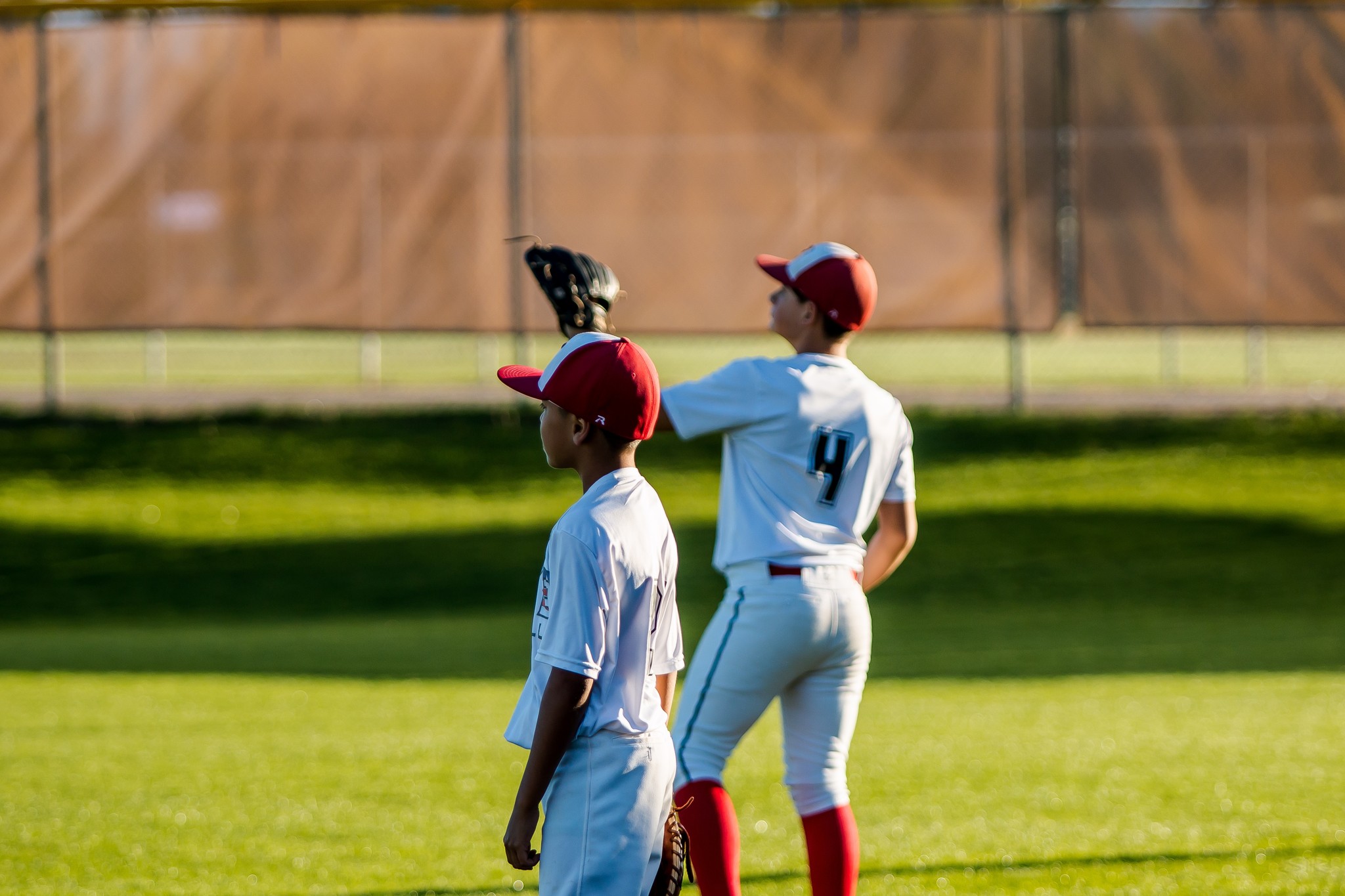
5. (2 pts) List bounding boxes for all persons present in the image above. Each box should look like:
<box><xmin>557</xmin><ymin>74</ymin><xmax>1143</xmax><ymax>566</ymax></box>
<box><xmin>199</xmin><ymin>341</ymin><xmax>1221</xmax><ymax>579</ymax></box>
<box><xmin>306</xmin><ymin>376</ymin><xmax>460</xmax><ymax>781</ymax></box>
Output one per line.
<box><xmin>522</xmin><ymin>242</ymin><xmax>919</xmax><ymax>896</ymax></box>
<box><xmin>497</xmin><ymin>330</ymin><xmax>685</xmax><ymax>896</ymax></box>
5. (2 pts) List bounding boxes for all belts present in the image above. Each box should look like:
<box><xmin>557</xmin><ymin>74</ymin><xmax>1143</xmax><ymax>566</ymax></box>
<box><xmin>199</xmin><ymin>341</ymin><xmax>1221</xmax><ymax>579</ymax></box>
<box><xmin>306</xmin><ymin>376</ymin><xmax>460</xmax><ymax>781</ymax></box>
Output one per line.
<box><xmin>763</xmin><ymin>560</ymin><xmax>802</xmax><ymax>578</ymax></box>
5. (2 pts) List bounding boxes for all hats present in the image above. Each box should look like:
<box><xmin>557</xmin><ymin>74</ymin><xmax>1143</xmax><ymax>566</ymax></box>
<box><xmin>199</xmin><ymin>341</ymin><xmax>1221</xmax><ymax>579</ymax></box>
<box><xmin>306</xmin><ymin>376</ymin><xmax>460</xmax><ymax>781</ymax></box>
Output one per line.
<box><xmin>752</xmin><ymin>242</ymin><xmax>877</xmax><ymax>331</ymax></box>
<box><xmin>496</xmin><ymin>332</ymin><xmax>661</xmax><ymax>440</ymax></box>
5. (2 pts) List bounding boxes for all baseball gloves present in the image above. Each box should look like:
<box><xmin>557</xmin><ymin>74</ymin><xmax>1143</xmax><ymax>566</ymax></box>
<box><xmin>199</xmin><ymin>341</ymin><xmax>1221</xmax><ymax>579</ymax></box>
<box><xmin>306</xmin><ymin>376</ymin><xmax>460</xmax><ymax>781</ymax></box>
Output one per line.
<box><xmin>502</xmin><ymin>232</ymin><xmax>628</xmax><ymax>338</ymax></box>
